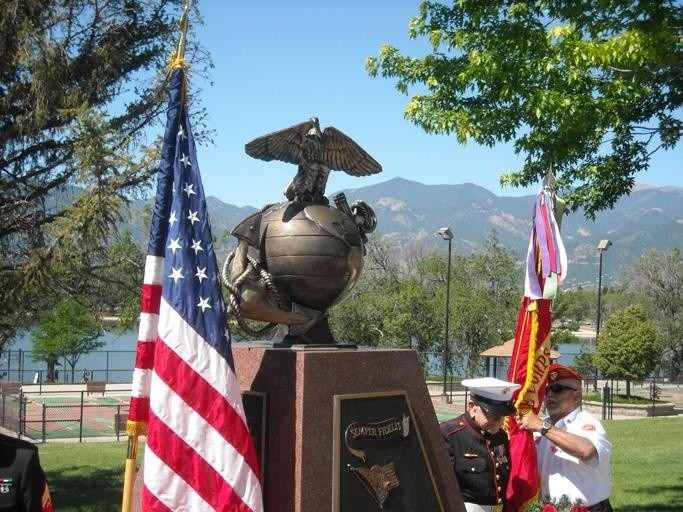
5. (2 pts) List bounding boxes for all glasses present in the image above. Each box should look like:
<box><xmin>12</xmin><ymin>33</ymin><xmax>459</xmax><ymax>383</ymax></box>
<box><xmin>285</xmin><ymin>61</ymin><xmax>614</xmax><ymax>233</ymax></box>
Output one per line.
<box><xmin>546</xmin><ymin>384</ymin><xmax>578</xmax><ymax>393</ymax></box>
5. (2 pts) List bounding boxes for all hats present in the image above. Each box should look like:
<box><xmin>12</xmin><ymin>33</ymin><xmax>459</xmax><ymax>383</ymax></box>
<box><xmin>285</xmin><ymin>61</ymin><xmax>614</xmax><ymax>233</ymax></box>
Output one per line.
<box><xmin>544</xmin><ymin>363</ymin><xmax>582</xmax><ymax>395</ymax></box>
<box><xmin>461</xmin><ymin>377</ymin><xmax>522</xmax><ymax>416</ymax></box>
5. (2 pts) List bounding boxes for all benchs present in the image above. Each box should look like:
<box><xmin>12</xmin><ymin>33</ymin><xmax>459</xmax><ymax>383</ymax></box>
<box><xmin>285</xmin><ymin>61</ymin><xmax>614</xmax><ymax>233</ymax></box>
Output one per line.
<box><xmin>86</xmin><ymin>381</ymin><xmax>105</xmax><ymax>396</ymax></box>
<box><xmin>115</xmin><ymin>414</ymin><xmax>130</xmax><ymax>436</ymax></box>
<box><xmin>1</xmin><ymin>382</ymin><xmax>22</xmax><ymax>395</ymax></box>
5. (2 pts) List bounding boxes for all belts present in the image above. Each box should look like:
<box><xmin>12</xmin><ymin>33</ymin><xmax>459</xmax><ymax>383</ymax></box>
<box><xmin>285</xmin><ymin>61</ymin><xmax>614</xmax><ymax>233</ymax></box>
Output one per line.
<box><xmin>587</xmin><ymin>498</ymin><xmax>610</xmax><ymax>510</ymax></box>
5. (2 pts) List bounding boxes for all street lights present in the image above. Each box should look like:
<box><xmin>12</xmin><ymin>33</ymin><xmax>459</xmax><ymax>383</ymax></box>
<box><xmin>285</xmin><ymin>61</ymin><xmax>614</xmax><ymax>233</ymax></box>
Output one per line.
<box><xmin>593</xmin><ymin>238</ymin><xmax>615</xmax><ymax>393</ymax></box>
<box><xmin>437</xmin><ymin>224</ymin><xmax>457</xmax><ymax>398</ymax></box>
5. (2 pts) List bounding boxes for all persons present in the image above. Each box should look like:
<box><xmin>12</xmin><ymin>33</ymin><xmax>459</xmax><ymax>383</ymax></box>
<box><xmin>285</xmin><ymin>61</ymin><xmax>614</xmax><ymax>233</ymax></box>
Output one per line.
<box><xmin>439</xmin><ymin>378</ymin><xmax>522</xmax><ymax>512</ymax></box>
<box><xmin>517</xmin><ymin>364</ymin><xmax>614</xmax><ymax>512</ymax></box>
<box><xmin>0</xmin><ymin>432</ymin><xmax>54</xmax><ymax>512</ymax></box>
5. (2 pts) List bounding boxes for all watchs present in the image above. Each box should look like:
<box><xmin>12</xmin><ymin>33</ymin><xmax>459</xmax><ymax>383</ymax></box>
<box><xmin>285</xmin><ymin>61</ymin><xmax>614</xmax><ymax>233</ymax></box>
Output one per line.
<box><xmin>541</xmin><ymin>421</ymin><xmax>552</xmax><ymax>435</ymax></box>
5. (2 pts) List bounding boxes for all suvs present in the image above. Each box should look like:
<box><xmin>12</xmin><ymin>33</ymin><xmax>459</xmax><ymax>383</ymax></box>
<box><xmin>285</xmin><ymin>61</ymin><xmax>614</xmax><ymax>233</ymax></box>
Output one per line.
<box><xmin>558</xmin><ymin>319</ymin><xmax>572</xmax><ymax>329</ymax></box>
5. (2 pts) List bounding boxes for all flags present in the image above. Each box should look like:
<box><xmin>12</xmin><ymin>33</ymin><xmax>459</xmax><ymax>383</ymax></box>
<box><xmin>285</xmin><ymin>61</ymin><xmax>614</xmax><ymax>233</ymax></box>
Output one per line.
<box><xmin>126</xmin><ymin>67</ymin><xmax>264</xmax><ymax>511</ymax></box>
<box><xmin>505</xmin><ymin>189</ymin><xmax>567</xmax><ymax>512</ymax></box>
